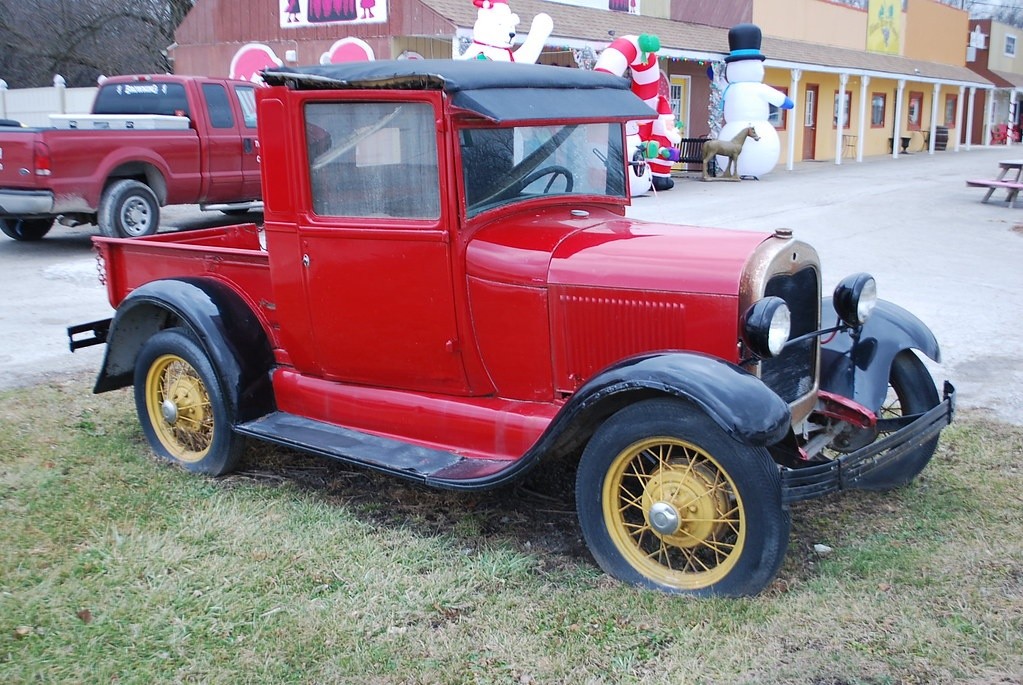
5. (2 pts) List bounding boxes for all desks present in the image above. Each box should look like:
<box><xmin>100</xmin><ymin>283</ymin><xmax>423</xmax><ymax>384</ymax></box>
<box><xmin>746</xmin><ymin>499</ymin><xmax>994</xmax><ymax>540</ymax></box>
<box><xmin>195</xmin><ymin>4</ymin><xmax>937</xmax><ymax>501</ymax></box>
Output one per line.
<box><xmin>919</xmin><ymin>130</ymin><xmax>930</xmax><ymax>152</ymax></box>
<box><xmin>979</xmin><ymin>159</ymin><xmax>1023</xmax><ymax>208</ymax></box>
<box><xmin>843</xmin><ymin>135</ymin><xmax>857</xmax><ymax>159</ymax></box>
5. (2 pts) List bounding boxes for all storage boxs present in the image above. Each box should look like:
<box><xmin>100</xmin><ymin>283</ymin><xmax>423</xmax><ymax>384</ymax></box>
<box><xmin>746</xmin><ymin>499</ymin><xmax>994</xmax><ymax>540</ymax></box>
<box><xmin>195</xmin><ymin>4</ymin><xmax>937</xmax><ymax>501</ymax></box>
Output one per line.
<box><xmin>49</xmin><ymin>113</ymin><xmax>192</xmax><ymax>131</ymax></box>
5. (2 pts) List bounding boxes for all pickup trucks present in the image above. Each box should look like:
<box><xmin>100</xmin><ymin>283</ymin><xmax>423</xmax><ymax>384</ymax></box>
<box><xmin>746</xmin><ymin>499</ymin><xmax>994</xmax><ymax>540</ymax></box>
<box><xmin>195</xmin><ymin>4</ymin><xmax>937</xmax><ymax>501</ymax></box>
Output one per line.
<box><xmin>64</xmin><ymin>60</ymin><xmax>958</xmax><ymax>600</ymax></box>
<box><xmin>0</xmin><ymin>72</ymin><xmax>332</xmax><ymax>243</ymax></box>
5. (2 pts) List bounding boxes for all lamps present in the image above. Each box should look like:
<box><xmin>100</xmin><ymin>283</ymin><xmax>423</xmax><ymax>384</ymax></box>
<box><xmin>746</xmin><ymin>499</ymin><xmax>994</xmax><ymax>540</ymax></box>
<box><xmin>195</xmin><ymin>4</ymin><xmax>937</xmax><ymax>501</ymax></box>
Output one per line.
<box><xmin>608</xmin><ymin>30</ymin><xmax>615</xmax><ymax>40</ymax></box>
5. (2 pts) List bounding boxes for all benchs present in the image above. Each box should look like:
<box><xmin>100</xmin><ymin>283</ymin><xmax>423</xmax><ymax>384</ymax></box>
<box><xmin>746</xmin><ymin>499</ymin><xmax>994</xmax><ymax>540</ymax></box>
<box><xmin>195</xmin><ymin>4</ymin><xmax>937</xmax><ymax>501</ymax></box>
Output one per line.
<box><xmin>967</xmin><ymin>178</ymin><xmax>1023</xmax><ymax>191</ymax></box>
<box><xmin>676</xmin><ymin>138</ymin><xmax>717</xmax><ymax>178</ymax></box>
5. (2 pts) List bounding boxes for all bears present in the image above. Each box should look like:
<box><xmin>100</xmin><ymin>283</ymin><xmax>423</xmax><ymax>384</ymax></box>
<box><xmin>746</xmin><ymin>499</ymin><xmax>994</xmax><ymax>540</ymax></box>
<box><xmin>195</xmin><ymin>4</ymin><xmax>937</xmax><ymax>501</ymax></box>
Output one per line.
<box><xmin>458</xmin><ymin>0</ymin><xmax>553</xmax><ymax>64</ymax></box>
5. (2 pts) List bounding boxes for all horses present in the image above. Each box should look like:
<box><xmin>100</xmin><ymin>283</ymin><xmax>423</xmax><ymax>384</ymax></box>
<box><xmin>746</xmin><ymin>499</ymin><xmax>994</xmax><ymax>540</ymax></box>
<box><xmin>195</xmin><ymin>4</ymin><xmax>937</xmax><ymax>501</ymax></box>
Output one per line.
<box><xmin>701</xmin><ymin>126</ymin><xmax>761</xmax><ymax>179</ymax></box>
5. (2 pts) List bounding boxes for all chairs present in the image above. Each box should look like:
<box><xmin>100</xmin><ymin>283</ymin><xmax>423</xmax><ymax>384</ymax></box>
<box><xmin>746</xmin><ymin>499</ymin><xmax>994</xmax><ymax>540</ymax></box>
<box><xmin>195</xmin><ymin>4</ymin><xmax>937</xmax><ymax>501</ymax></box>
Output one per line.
<box><xmin>991</xmin><ymin>124</ymin><xmax>1020</xmax><ymax>146</ymax></box>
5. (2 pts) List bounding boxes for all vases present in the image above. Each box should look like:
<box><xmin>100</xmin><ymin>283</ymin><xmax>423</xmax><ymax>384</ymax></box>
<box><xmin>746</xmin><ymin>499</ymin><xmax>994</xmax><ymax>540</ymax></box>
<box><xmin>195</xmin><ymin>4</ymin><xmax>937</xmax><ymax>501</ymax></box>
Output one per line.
<box><xmin>889</xmin><ymin>137</ymin><xmax>893</xmax><ymax>154</ymax></box>
<box><xmin>900</xmin><ymin>137</ymin><xmax>911</xmax><ymax>155</ymax></box>
